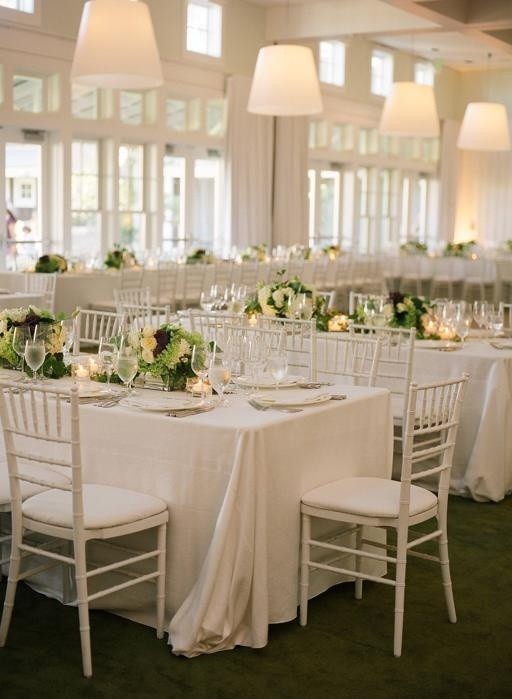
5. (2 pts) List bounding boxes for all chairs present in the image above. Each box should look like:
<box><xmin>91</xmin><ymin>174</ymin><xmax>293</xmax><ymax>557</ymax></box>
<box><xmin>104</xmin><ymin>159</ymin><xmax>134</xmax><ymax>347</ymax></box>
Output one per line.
<box><xmin>222</xmin><ymin>324</ymin><xmax>286</xmax><ymax>375</ymax></box>
<box><xmin>119</xmin><ymin>305</ymin><xmax>172</xmax><ymax>342</ymax></box>
<box><xmin>348</xmin><ymin>290</ymin><xmax>384</xmax><ymax>314</ymax></box>
<box><xmin>346</xmin><ymin>318</ymin><xmax>448</xmax><ymax>466</ymax></box>
<box><xmin>112</xmin><ymin>288</ymin><xmax>155</xmax><ymax>316</ymax></box>
<box><xmin>29</xmin><ymin>273</ymin><xmax>59</xmax><ymax>317</ymax></box>
<box><xmin>298</xmin><ymin>378</ymin><xmax>469</xmax><ymax>657</ymax></box>
<box><xmin>313</xmin><ymin>333</ymin><xmax>382</xmax><ymax>386</ymax></box>
<box><xmin>312</xmin><ymin>287</ymin><xmax>334</xmax><ymax>313</ymax></box>
<box><xmin>187</xmin><ymin>309</ymin><xmax>246</xmax><ymax>363</ymax></box>
<box><xmin>72</xmin><ymin>305</ymin><xmax>124</xmax><ymax>359</ymax></box>
<box><xmin>498</xmin><ymin>302</ymin><xmax>512</xmax><ymax>325</ymax></box>
<box><xmin>0</xmin><ymin>381</ymin><xmax>166</xmax><ymax>677</ymax></box>
<box><xmin>239</xmin><ymin>316</ymin><xmax>318</xmax><ymax>372</ymax></box>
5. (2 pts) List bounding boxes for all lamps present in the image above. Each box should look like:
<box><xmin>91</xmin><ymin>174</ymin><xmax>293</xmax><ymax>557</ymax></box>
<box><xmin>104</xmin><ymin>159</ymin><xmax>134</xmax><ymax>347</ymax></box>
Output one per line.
<box><xmin>248</xmin><ymin>45</ymin><xmax>327</xmax><ymax>115</ymax></box>
<box><xmin>376</xmin><ymin>79</ymin><xmax>439</xmax><ymax>140</ymax></box>
<box><xmin>72</xmin><ymin>0</ymin><xmax>165</xmax><ymax>89</ymax></box>
<box><xmin>455</xmin><ymin>100</ymin><xmax>509</xmax><ymax>152</ymax></box>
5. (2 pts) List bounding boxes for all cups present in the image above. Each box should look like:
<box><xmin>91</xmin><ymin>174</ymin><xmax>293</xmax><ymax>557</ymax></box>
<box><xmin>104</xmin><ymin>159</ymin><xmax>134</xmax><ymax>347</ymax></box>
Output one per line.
<box><xmin>199</xmin><ymin>291</ymin><xmax>215</xmax><ymax>317</ymax></box>
<box><xmin>96</xmin><ymin>336</ymin><xmax>119</xmax><ymax>397</ymax></box>
<box><xmin>433</xmin><ymin>297</ymin><xmax>471</xmax><ymax>346</ymax></box>
<box><xmin>471</xmin><ymin>299</ymin><xmax>492</xmax><ymax>344</ymax></box>
<box><xmin>231</xmin><ymin>284</ymin><xmax>246</xmax><ymax>319</ymax></box>
<box><xmin>286</xmin><ymin>293</ymin><xmax>307</xmax><ymax>328</ymax></box>
<box><xmin>489</xmin><ymin>310</ymin><xmax>504</xmax><ymax>342</ymax></box>
<box><xmin>481</xmin><ymin>302</ymin><xmax>494</xmax><ymax>342</ymax></box>
<box><xmin>33</xmin><ymin>323</ymin><xmax>54</xmax><ymax>382</ymax></box>
<box><xmin>118</xmin><ymin>334</ymin><xmax>141</xmax><ymax>390</ymax></box>
<box><xmin>190</xmin><ymin>343</ymin><xmax>214</xmax><ymax>396</ymax></box>
<box><xmin>240</xmin><ymin>334</ymin><xmax>265</xmax><ymax>391</ymax></box>
<box><xmin>210</xmin><ymin>282</ymin><xmax>229</xmax><ymax>319</ymax></box>
<box><xmin>209</xmin><ymin>359</ymin><xmax>232</xmax><ymax>407</ymax></box>
<box><xmin>111</xmin><ymin>352</ymin><xmax>139</xmax><ymax>398</ymax></box>
<box><xmin>59</xmin><ymin>320</ymin><xmax>78</xmax><ymax>357</ymax></box>
<box><xmin>23</xmin><ymin>337</ymin><xmax>47</xmax><ymax>387</ymax></box>
<box><xmin>12</xmin><ymin>325</ymin><xmax>34</xmax><ymax>384</ymax></box>
<box><xmin>224</xmin><ymin>334</ymin><xmax>247</xmax><ymax>390</ymax></box>
<box><xmin>268</xmin><ymin>352</ymin><xmax>290</xmax><ymax>393</ymax></box>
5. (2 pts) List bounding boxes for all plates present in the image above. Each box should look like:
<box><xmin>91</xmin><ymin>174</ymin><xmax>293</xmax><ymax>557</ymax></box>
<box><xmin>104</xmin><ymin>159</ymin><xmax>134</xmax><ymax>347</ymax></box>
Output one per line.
<box><xmin>232</xmin><ymin>374</ymin><xmax>308</xmax><ymax>388</ymax></box>
<box><xmin>407</xmin><ymin>339</ymin><xmax>466</xmax><ymax>349</ymax></box>
<box><xmin>36</xmin><ymin>378</ymin><xmax>112</xmax><ymax>399</ymax></box>
<box><xmin>120</xmin><ymin>391</ymin><xmax>205</xmax><ymax>412</ymax></box>
<box><xmin>248</xmin><ymin>387</ymin><xmax>333</xmax><ymax>411</ymax></box>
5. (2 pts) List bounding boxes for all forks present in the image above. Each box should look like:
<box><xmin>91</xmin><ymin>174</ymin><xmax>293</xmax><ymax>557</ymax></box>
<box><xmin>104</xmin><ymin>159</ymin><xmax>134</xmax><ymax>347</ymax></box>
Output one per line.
<box><xmin>490</xmin><ymin>341</ymin><xmax>511</xmax><ymax>350</ymax></box>
<box><xmin>248</xmin><ymin>398</ymin><xmax>303</xmax><ymax>413</ymax></box>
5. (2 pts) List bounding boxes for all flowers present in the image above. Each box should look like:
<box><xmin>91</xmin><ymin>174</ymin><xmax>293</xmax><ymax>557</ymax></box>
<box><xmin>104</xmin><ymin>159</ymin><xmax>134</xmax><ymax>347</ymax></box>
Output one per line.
<box><xmin>137</xmin><ymin>325</ymin><xmax>205</xmax><ymax>387</ymax></box>
<box><xmin>0</xmin><ymin>306</ymin><xmax>63</xmax><ymax>366</ymax></box>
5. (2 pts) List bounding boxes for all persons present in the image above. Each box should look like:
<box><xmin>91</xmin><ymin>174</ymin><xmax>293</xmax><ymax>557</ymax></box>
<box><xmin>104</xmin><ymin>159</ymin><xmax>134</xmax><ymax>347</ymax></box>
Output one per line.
<box><xmin>20</xmin><ymin>226</ymin><xmax>38</xmax><ymax>260</ymax></box>
<box><xmin>6</xmin><ymin>209</ymin><xmax>19</xmax><ymax>260</ymax></box>
